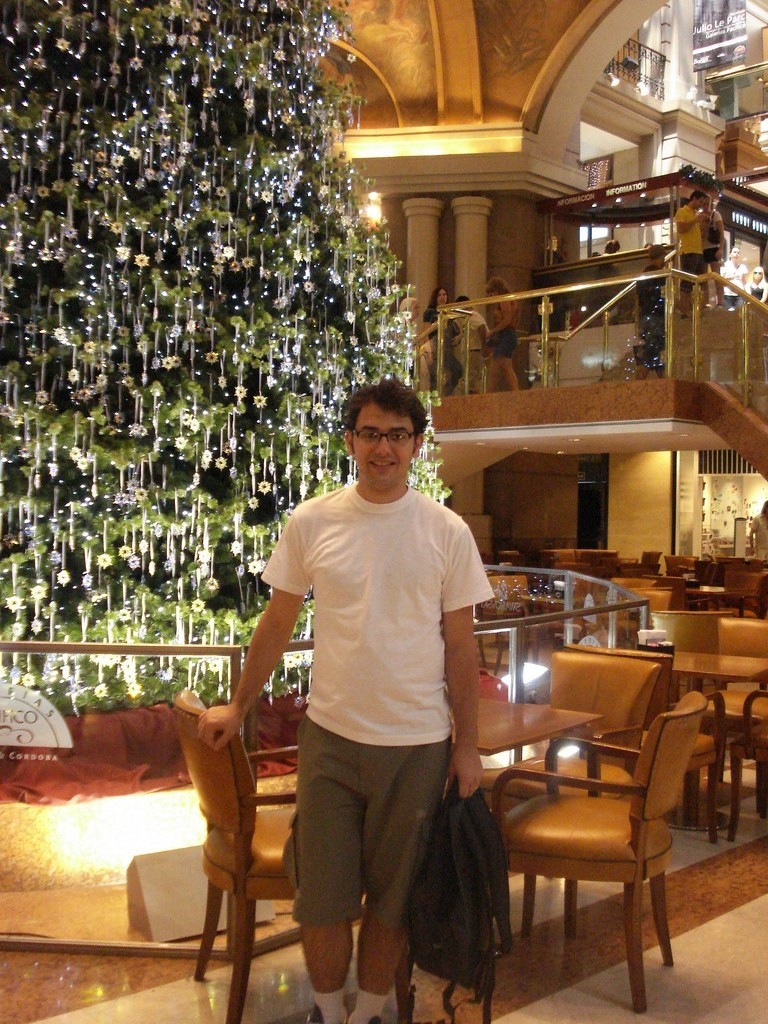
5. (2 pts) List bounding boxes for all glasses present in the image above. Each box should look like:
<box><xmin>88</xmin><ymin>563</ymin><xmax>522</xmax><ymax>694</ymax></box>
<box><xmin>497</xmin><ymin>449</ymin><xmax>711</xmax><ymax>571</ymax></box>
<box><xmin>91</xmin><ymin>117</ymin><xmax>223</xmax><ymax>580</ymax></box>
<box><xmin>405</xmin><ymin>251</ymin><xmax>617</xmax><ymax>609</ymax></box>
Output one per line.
<box><xmin>351</xmin><ymin>425</ymin><xmax>417</xmax><ymax>447</ymax></box>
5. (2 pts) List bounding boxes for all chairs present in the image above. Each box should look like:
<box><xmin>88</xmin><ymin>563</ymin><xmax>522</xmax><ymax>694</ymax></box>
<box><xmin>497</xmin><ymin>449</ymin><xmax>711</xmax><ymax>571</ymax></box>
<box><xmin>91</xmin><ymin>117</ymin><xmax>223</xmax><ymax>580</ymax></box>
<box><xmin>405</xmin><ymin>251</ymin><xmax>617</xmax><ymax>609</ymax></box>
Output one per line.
<box><xmin>472</xmin><ymin>538</ymin><xmax>768</xmax><ymax>847</ymax></box>
<box><xmin>173</xmin><ymin>690</ymin><xmax>413</xmax><ymax>1024</ymax></box>
<box><xmin>492</xmin><ymin>690</ymin><xmax>707</xmax><ymax>1012</ymax></box>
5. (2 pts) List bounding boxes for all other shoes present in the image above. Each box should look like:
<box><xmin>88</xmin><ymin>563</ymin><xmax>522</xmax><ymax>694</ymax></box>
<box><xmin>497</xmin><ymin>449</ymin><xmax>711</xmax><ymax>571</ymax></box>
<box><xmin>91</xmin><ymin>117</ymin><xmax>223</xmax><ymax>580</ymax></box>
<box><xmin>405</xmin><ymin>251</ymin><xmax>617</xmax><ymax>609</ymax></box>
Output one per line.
<box><xmin>307</xmin><ymin>1003</ymin><xmax>324</xmax><ymax>1024</ymax></box>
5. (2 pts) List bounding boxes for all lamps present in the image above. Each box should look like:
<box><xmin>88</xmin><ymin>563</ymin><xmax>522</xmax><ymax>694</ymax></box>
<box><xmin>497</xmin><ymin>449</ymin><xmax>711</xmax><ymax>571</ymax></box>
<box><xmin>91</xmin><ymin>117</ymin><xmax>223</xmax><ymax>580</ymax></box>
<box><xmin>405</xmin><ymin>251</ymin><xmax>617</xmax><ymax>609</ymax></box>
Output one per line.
<box><xmin>633</xmin><ymin>83</ymin><xmax>648</xmax><ymax>95</ymax></box>
<box><xmin>696</xmin><ymin>100</ymin><xmax>716</xmax><ymax>111</ymax></box>
<box><xmin>621</xmin><ymin>56</ymin><xmax>639</xmax><ymax>72</ymax></box>
<box><xmin>126</xmin><ymin>846</ymin><xmax>276</xmax><ymax>944</ymax></box>
<box><xmin>605</xmin><ymin>73</ymin><xmax>620</xmax><ymax>87</ymax></box>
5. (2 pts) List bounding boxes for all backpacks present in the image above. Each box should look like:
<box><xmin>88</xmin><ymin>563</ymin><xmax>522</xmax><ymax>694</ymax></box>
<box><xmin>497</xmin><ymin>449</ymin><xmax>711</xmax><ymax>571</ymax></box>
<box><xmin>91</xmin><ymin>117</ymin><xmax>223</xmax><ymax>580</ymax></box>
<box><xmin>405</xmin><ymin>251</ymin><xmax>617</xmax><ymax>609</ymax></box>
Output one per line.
<box><xmin>402</xmin><ymin>777</ymin><xmax>505</xmax><ymax>988</ymax></box>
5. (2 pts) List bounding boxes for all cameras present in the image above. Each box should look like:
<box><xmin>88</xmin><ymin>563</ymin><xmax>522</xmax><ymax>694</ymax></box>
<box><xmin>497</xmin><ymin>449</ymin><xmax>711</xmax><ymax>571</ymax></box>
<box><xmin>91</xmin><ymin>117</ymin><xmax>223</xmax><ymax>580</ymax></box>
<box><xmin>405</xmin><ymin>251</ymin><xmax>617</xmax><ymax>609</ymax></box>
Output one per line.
<box><xmin>705</xmin><ymin>216</ymin><xmax>710</xmax><ymax>222</ymax></box>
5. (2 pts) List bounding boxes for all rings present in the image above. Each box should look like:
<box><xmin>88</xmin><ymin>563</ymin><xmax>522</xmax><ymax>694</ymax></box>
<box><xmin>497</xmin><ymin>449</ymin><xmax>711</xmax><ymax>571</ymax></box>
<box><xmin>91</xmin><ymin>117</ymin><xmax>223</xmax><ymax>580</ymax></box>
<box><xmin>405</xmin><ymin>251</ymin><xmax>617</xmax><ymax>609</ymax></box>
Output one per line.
<box><xmin>473</xmin><ymin>785</ymin><xmax>479</xmax><ymax>791</ymax></box>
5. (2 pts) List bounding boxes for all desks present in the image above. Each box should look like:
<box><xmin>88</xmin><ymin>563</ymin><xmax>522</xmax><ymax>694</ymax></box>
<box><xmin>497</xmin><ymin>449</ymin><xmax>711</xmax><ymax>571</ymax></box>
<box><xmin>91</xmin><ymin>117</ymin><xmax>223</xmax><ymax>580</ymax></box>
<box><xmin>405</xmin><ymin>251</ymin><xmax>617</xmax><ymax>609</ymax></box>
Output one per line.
<box><xmin>545</xmin><ymin>548</ymin><xmax>618</xmax><ymax>563</ymax></box>
<box><xmin>450</xmin><ymin>698</ymin><xmax>604</xmax><ymax>755</ymax></box>
<box><xmin>684</xmin><ymin>585</ymin><xmax>756</xmax><ymax>613</ymax></box>
<box><xmin>664</xmin><ymin>651</ymin><xmax>768</xmax><ymax>831</ymax></box>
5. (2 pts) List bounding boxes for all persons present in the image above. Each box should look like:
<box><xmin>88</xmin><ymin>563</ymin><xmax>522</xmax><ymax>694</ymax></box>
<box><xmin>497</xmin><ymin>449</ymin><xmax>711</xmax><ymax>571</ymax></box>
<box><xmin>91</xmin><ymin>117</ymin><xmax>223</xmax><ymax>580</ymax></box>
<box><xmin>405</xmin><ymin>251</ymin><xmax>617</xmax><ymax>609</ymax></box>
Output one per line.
<box><xmin>634</xmin><ymin>245</ymin><xmax>665</xmax><ymax>378</ymax></box>
<box><xmin>720</xmin><ymin>245</ymin><xmax>748</xmax><ymax>312</ymax></box>
<box><xmin>195</xmin><ymin>381</ymin><xmax>495</xmax><ymax>1024</ymax></box>
<box><xmin>605</xmin><ymin>240</ymin><xmax>620</xmax><ymax>254</ymax></box>
<box><xmin>592</xmin><ymin>251</ymin><xmax>602</xmax><ymax>257</ymax></box>
<box><xmin>392</xmin><ymin>276</ymin><xmax>522</xmax><ymax>394</ymax></box>
<box><xmin>749</xmin><ymin>500</ymin><xmax>768</xmax><ymax>561</ymax></box>
<box><xmin>674</xmin><ymin>190</ymin><xmax>723</xmax><ymax>318</ymax></box>
<box><xmin>745</xmin><ymin>266</ymin><xmax>768</xmax><ymax>303</ymax></box>
